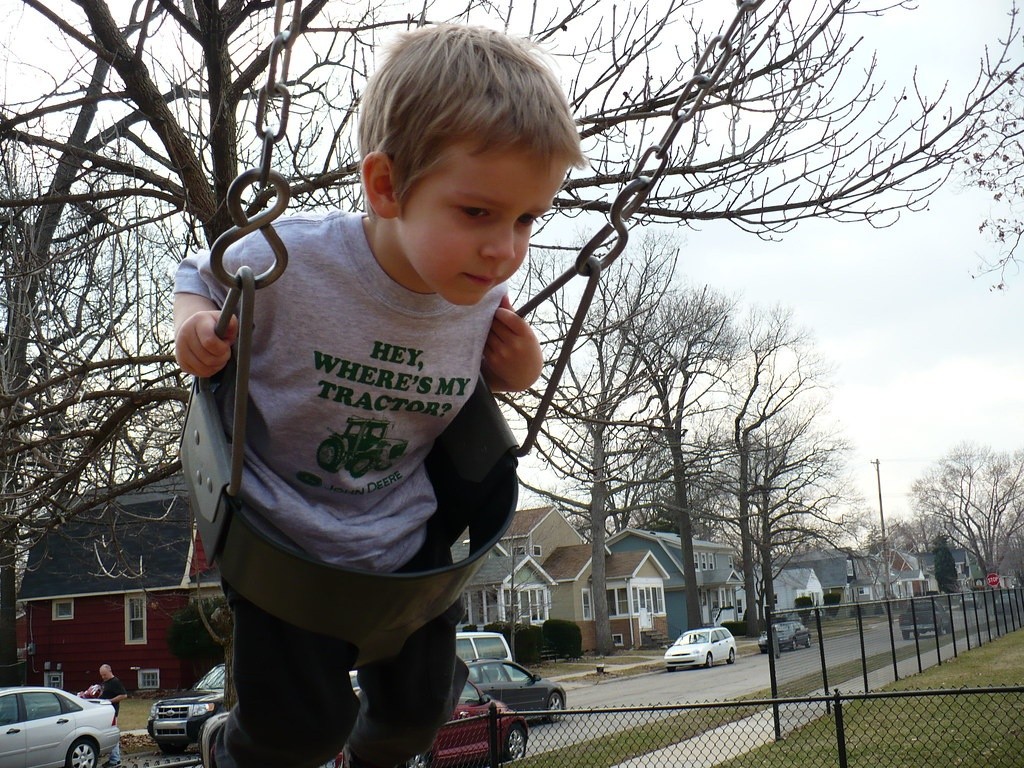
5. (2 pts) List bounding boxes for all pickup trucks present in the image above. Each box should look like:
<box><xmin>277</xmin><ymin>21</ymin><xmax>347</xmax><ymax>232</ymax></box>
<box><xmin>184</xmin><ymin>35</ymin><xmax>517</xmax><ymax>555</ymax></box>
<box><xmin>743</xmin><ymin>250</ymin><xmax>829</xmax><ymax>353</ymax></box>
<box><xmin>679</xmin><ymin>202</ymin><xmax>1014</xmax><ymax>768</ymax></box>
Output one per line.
<box><xmin>899</xmin><ymin>601</ymin><xmax>951</xmax><ymax>640</ymax></box>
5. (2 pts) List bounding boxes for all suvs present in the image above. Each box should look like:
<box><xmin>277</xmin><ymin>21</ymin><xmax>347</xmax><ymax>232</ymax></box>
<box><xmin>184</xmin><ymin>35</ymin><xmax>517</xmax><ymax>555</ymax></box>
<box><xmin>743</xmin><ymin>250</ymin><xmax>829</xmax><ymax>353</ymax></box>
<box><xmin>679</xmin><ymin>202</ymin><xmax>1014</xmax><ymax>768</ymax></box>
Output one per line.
<box><xmin>146</xmin><ymin>664</ymin><xmax>228</xmax><ymax>756</ymax></box>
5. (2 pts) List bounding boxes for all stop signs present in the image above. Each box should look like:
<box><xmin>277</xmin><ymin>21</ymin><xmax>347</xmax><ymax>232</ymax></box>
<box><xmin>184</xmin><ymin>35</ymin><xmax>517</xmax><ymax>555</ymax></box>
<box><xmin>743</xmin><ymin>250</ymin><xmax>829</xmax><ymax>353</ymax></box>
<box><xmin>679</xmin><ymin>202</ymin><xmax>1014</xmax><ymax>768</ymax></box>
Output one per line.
<box><xmin>986</xmin><ymin>574</ymin><xmax>1000</xmax><ymax>587</ymax></box>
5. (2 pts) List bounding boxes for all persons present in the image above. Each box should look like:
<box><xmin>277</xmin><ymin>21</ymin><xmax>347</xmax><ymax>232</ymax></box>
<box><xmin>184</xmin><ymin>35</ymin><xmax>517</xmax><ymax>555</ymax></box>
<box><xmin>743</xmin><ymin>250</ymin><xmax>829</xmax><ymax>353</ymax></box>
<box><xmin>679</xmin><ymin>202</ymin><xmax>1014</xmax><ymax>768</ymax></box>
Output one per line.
<box><xmin>98</xmin><ymin>664</ymin><xmax>127</xmax><ymax>768</ymax></box>
<box><xmin>173</xmin><ymin>21</ymin><xmax>589</xmax><ymax>768</ymax></box>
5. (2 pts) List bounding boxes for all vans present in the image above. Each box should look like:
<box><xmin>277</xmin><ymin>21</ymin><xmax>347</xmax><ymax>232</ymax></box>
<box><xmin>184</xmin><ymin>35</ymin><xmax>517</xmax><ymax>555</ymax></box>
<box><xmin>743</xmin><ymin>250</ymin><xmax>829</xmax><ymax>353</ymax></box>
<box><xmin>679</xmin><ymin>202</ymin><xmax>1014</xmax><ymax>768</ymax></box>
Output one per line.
<box><xmin>456</xmin><ymin>631</ymin><xmax>512</xmax><ymax>685</ymax></box>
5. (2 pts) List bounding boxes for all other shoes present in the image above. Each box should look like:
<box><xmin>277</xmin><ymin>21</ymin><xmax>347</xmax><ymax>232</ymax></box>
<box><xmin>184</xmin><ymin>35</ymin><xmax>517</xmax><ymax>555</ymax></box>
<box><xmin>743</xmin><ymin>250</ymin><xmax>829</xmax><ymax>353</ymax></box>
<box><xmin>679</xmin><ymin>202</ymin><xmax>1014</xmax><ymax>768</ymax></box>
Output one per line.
<box><xmin>102</xmin><ymin>761</ymin><xmax>122</xmax><ymax>768</ymax></box>
<box><xmin>198</xmin><ymin>711</ymin><xmax>231</xmax><ymax>768</ymax></box>
<box><xmin>340</xmin><ymin>687</ymin><xmax>362</xmax><ymax>768</ymax></box>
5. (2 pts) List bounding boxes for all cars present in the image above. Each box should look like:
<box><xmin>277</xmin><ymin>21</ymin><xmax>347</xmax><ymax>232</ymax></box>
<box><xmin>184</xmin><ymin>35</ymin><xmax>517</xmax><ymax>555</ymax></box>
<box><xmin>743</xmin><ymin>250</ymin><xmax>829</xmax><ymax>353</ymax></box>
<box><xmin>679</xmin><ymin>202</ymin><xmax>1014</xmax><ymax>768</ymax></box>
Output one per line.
<box><xmin>318</xmin><ymin>678</ymin><xmax>529</xmax><ymax>768</ymax></box>
<box><xmin>959</xmin><ymin>593</ymin><xmax>982</xmax><ymax>611</ymax></box>
<box><xmin>664</xmin><ymin>626</ymin><xmax>737</xmax><ymax>672</ymax></box>
<box><xmin>993</xmin><ymin>593</ymin><xmax>1021</xmax><ymax>614</ymax></box>
<box><xmin>0</xmin><ymin>686</ymin><xmax>120</xmax><ymax>768</ymax></box>
<box><xmin>463</xmin><ymin>657</ymin><xmax>567</xmax><ymax>723</ymax></box>
<box><xmin>758</xmin><ymin>621</ymin><xmax>811</xmax><ymax>654</ymax></box>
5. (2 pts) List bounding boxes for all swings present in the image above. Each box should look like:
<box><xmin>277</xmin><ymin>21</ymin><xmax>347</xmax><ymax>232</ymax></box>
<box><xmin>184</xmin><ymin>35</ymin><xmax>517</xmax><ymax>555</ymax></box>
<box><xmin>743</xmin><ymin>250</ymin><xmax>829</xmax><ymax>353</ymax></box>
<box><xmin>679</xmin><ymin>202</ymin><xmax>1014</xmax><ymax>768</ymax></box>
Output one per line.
<box><xmin>178</xmin><ymin>1</ymin><xmax>768</xmax><ymax>674</ymax></box>
